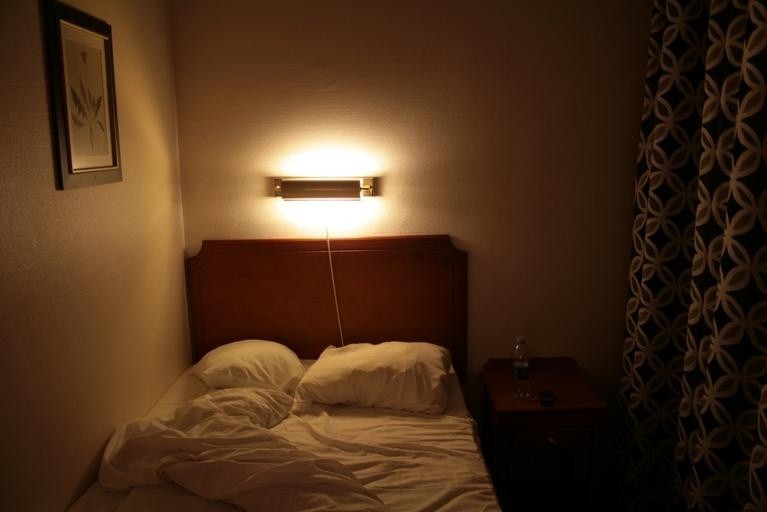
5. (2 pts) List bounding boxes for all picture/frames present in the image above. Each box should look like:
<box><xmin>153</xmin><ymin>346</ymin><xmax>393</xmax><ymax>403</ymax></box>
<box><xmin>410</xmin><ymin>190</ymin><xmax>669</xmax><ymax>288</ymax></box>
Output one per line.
<box><xmin>38</xmin><ymin>0</ymin><xmax>123</xmax><ymax>191</ymax></box>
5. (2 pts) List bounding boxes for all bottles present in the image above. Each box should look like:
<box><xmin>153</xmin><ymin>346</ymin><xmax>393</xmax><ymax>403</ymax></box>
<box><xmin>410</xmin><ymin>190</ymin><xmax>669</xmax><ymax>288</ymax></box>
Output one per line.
<box><xmin>511</xmin><ymin>336</ymin><xmax>531</xmax><ymax>401</ymax></box>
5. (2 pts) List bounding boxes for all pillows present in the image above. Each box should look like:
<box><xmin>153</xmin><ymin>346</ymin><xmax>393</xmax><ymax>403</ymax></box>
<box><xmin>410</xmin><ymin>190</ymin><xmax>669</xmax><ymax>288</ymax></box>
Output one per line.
<box><xmin>193</xmin><ymin>340</ymin><xmax>306</xmax><ymax>396</ymax></box>
<box><xmin>289</xmin><ymin>342</ymin><xmax>451</xmax><ymax>415</ymax></box>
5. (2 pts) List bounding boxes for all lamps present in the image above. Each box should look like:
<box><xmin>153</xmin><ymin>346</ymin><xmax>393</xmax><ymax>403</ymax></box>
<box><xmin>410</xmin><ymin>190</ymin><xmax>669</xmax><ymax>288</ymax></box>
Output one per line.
<box><xmin>273</xmin><ymin>176</ymin><xmax>376</xmax><ymax>201</ymax></box>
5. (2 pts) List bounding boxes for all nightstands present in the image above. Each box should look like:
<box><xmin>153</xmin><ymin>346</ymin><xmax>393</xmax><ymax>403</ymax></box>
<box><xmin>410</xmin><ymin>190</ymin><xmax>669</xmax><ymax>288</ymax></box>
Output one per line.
<box><xmin>486</xmin><ymin>357</ymin><xmax>588</xmax><ymax>445</ymax></box>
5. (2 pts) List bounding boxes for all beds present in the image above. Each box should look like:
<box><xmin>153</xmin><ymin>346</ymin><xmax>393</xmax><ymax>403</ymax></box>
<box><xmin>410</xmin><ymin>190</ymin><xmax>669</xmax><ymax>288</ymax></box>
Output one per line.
<box><xmin>68</xmin><ymin>234</ymin><xmax>502</xmax><ymax>512</ymax></box>
